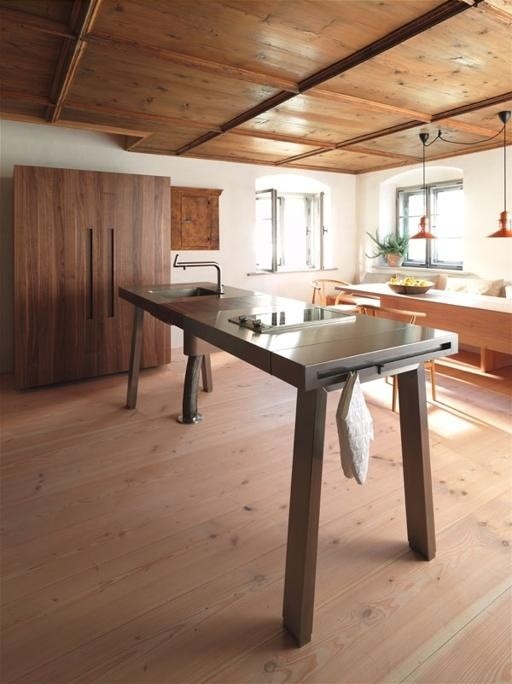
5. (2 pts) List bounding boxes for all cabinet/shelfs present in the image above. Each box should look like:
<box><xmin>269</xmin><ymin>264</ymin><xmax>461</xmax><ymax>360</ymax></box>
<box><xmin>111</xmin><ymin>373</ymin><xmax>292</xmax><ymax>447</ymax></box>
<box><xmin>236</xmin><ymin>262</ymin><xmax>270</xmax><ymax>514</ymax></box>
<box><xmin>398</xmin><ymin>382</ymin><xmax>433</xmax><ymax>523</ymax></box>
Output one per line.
<box><xmin>13</xmin><ymin>163</ymin><xmax>170</xmax><ymax>389</ymax></box>
<box><xmin>171</xmin><ymin>184</ymin><xmax>224</xmax><ymax>250</ymax></box>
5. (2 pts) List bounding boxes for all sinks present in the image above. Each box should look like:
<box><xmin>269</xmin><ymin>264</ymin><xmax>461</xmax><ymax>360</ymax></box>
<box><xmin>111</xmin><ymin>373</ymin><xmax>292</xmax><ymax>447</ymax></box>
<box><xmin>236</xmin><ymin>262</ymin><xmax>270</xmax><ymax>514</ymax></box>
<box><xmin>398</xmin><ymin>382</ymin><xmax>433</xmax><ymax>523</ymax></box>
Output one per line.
<box><xmin>146</xmin><ymin>287</ymin><xmax>218</xmax><ymax>299</ymax></box>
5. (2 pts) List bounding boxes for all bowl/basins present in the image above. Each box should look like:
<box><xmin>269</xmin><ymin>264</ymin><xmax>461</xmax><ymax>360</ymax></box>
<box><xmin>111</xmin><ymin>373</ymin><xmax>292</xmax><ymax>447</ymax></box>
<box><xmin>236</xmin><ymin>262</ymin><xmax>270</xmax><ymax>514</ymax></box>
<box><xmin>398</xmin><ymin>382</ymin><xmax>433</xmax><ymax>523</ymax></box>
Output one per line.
<box><xmin>387</xmin><ymin>280</ymin><xmax>434</xmax><ymax>294</ymax></box>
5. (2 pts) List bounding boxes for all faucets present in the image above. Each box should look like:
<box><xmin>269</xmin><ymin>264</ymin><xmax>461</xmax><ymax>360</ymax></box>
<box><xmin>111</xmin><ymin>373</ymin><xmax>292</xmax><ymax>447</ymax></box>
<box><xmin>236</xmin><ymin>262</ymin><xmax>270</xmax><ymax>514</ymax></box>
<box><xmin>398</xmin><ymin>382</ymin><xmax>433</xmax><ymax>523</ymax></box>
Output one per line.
<box><xmin>213</xmin><ymin>309</ymin><xmax>221</xmax><ymax>328</ymax></box>
<box><xmin>173</xmin><ymin>254</ymin><xmax>222</xmax><ymax>293</ymax></box>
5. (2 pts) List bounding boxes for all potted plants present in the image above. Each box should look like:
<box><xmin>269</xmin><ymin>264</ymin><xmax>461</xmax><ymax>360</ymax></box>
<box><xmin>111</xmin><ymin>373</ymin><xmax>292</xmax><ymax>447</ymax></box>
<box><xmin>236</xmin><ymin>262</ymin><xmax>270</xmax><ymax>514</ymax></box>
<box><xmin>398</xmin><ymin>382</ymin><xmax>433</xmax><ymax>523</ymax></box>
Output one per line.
<box><xmin>363</xmin><ymin>229</ymin><xmax>411</xmax><ymax>266</ymax></box>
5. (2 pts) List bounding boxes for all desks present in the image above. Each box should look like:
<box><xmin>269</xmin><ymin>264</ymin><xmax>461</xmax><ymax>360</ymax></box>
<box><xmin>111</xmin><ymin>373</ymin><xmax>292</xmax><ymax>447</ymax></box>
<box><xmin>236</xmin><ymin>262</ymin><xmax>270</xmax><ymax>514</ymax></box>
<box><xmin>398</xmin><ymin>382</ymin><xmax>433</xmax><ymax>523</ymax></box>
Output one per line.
<box><xmin>118</xmin><ymin>281</ymin><xmax>460</xmax><ymax>644</ymax></box>
<box><xmin>332</xmin><ymin>280</ymin><xmax>512</xmax><ymax>373</ymax></box>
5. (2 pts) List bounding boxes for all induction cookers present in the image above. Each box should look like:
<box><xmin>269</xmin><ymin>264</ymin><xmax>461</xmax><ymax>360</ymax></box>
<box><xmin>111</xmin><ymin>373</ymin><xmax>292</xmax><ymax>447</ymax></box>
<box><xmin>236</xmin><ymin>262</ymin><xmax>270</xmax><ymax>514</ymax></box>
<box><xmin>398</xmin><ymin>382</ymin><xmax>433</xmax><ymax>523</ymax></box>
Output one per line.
<box><xmin>227</xmin><ymin>304</ymin><xmax>358</xmax><ymax>333</ymax></box>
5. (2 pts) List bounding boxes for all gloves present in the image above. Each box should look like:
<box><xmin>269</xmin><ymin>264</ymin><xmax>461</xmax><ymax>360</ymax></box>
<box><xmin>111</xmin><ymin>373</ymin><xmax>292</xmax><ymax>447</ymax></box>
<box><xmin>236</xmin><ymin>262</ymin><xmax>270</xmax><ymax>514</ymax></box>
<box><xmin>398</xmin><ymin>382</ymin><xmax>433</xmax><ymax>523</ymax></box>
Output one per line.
<box><xmin>335</xmin><ymin>372</ymin><xmax>374</xmax><ymax>485</ymax></box>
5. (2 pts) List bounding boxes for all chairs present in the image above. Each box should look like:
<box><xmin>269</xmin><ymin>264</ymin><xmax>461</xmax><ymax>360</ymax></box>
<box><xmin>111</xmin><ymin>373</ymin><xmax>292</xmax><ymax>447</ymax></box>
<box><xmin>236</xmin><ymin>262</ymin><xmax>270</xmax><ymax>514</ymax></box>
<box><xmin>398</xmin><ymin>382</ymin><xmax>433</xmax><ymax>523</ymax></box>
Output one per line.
<box><xmin>327</xmin><ymin>269</ymin><xmax>512</xmax><ymax>316</ymax></box>
<box><xmin>307</xmin><ymin>276</ymin><xmax>359</xmax><ymax>312</ymax></box>
<box><xmin>357</xmin><ymin>303</ymin><xmax>437</xmax><ymax>410</ymax></box>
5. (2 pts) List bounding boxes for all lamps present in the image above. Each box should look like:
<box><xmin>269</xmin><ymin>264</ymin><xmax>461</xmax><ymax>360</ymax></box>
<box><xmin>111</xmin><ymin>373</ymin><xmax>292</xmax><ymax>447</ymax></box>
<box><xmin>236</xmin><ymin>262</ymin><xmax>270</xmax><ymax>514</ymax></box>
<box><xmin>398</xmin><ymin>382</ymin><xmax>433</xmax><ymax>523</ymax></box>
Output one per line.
<box><xmin>408</xmin><ymin>111</ymin><xmax>511</xmax><ymax>240</ymax></box>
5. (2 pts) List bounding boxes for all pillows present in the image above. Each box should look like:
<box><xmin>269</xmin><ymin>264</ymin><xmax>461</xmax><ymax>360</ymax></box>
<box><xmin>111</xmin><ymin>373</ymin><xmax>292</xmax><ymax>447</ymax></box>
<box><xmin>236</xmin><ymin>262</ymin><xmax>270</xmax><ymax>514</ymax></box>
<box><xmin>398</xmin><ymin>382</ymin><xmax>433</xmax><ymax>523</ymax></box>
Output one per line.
<box><xmin>461</xmin><ymin>276</ymin><xmax>505</xmax><ymax>297</ymax></box>
